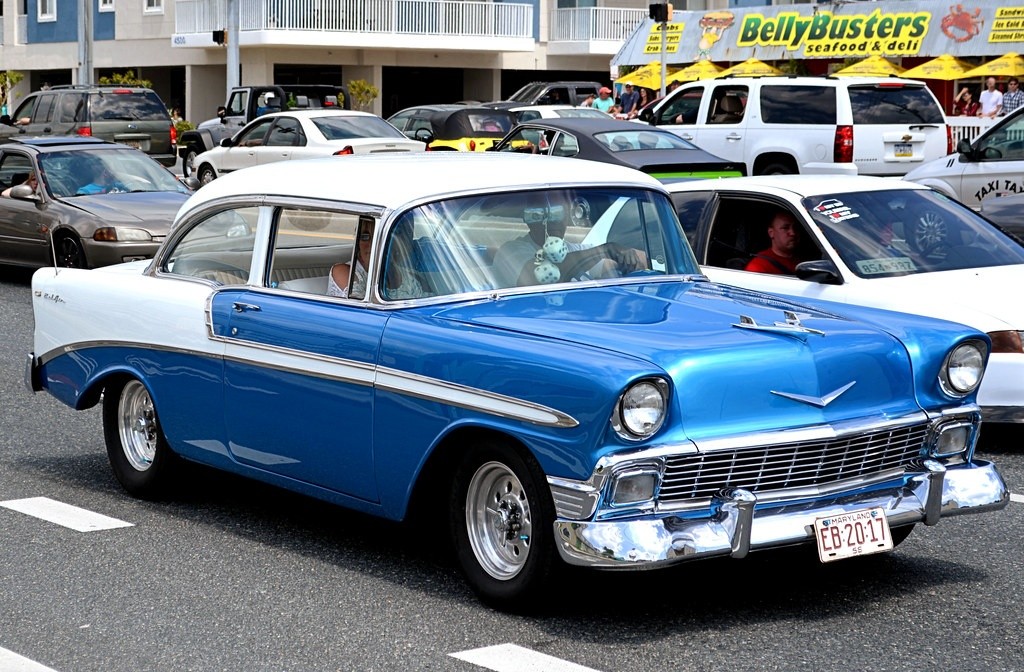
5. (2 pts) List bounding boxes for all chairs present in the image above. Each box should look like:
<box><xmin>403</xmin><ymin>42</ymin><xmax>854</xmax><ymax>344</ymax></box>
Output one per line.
<box><xmin>715</xmin><ymin>95</ymin><xmax>743</xmax><ymax>121</ymax></box>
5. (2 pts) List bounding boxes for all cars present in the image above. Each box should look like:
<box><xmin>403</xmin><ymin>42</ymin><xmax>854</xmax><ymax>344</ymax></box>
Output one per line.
<box><xmin>435</xmin><ymin>118</ymin><xmax>747</xmax><ymax>226</ymax></box>
<box><xmin>190</xmin><ymin>109</ymin><xmax>432</xmax><ymax>192</ymax></box>
<box><xmin>24</xmin><ymin>151</ymin><xmax>1009</xmax><ymax>610</ymax></box>
<box><xmin>895</xmin><ymin>104</ymin><xmax>1023</xmax><ymax>264</ymax></box>
<box><xmin>507</xmin><ymin>80</ymin><xmax>602</xmax><ymax>105</ymax></box>
<box><xmin>0</xmin><ymin>136</ymin><xmax>253</xmax><ymax>269</ymax></box>
<box><xmin>580</xmin><ymin>173</ymin><xmax>1024</xmax><ymax>425</ymax></box>
<box><xmin>178</xmin><ymin>84</ymin><xmax>350</xmax><ymax>180</ymax></box>
<box><xmin>385</xmin><ymin>100</ymin><xmax>617</xmax><ymax>154</ymax></box>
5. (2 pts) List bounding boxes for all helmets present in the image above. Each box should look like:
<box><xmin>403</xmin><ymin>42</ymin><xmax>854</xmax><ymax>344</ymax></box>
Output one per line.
<box><xmin>264</xmin><ymin>92</ymin><xmax>274</xmax><ymax>104</ymax></box>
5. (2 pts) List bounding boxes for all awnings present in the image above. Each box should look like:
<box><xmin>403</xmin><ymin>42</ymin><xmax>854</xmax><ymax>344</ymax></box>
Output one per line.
<box><xmin>614</xmin><ymin>52</ymin><xmax>1024</xmax><ymax>114</ymax></box>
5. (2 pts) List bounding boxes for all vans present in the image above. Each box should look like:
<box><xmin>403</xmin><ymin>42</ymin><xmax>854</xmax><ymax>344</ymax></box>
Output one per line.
<box><xmin>607</xmin><ymin>73</ymin><xmax>954</xmax><ymax>223</ymax></box>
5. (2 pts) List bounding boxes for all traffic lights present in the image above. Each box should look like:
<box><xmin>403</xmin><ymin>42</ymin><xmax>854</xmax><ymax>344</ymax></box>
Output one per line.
<box><xmin>213</xmin><ymin>30</ymin><xmax>227</xmax><ymax>45</ymax></box>
<box><xmin>648</xmin><ymin>3</ymin><xmax>673</xmax><ymax>22</ymax></box>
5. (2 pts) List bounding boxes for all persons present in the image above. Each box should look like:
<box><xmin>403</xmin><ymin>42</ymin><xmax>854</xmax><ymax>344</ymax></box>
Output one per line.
<box><xmin>744</xmin><ymin>200</ymin><xmax>930</xmax><ymax>275</ymax></box>
<box><xmin>954</xmin><ymin>77</ymin><xmax>1024</xmax><ymax>142</ymax></box>
<box><xmin>239</xmin><ymin>139</ymin><xmax>263</xmax><ymax>146</ymax></box>
<box><xmin>326</xmin><ymin>192</ymin><xmax>646</xmax><ymax>302</ymax></box>
<box><xmin>13</xmin><ymin>99</ymin><xmax>37</xmax><ymax>124</ymax></box>
<box><xmin>527</xmin><ymin>82</ymin><xmax>699</xmax><ymax>155</ymax></box>
<box><xmin>2</xmin><ymin>151</ymin><xmax>145</xmax><ymax>199</ymax></box>
<box><xmin>170</xmin><ymin>108</ymin><xmax>183</xmax><ymax>127</ymax></box>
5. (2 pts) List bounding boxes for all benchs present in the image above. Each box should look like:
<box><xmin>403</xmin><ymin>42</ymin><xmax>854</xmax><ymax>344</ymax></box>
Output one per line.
<box><xmin>171</xmin><ymin>245</ymin><xmax>353</xmax><ymax>286</ymax></box>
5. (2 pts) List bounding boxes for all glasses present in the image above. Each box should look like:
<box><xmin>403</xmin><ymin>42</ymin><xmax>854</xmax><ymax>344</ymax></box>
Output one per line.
<box><xmin>626</xmin><ymin>86</ymin><xmax>631</xmax><ymax>88</ymax></box>
<box><xmin>1010</xmin><ymin>83</ymin><xmax>1017</xmax><ymax>85</ymax></box>
<box><xmin>360</xmin><ymin>235</ymin><xmax>371</xmax><ymax>241</ymax></box>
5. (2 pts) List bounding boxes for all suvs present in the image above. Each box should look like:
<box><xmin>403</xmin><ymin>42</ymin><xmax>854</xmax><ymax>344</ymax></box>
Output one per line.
<box><xmin>0</xmin><ymin>84</ymin><xmax>178</xmax><ymax>167</ymax></box>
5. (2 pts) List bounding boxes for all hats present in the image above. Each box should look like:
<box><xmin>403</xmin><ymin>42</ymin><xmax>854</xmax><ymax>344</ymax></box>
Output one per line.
<box><xmin>600</xmin><ymin>88</ymin><xmax>611</xmax><ymax>93</ymax></box>
<box><xmin>625</xmin><ymin>82</ymin><xmax>632</xmax><ymax>85</ymax></box>
